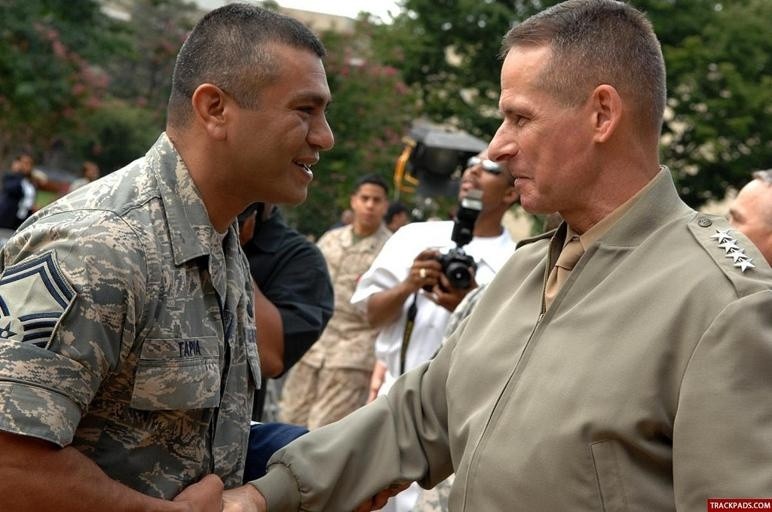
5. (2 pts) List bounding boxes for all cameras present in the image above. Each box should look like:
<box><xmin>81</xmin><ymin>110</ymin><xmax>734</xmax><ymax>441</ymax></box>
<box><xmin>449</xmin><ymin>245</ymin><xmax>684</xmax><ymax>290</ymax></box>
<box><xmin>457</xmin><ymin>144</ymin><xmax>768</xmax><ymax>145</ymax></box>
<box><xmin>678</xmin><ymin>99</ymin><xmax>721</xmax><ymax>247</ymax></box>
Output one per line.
<box><xmin>423</xmin><ymin>189</ymin><xmax>482</xmax><ymax>296</ymax></box>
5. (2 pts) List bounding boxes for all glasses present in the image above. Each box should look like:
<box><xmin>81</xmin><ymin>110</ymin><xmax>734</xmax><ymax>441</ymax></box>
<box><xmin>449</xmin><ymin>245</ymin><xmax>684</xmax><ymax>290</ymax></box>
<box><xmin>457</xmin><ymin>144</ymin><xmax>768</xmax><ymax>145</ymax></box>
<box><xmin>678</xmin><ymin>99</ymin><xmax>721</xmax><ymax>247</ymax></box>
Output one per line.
<box><xmin>465</xmin><ymin>156</ymin><xmax>503</xmax><ymax>176</ymax></box>
